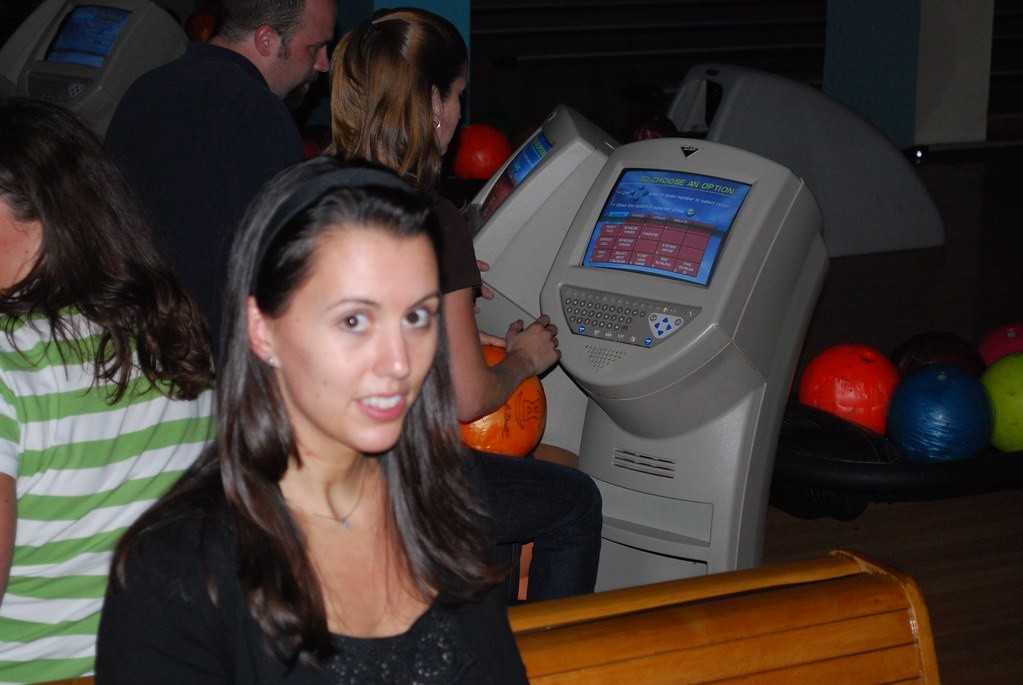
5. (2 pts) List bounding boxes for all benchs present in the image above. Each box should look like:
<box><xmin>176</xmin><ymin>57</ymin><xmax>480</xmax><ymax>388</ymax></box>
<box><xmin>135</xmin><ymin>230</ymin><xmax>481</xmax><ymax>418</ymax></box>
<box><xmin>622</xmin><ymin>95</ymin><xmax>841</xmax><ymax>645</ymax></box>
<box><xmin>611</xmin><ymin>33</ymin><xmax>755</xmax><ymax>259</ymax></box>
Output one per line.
<box><xmin>18</xmin><ymin>548</ymin><xmax>941</xmax><ymax>685</ymax></box>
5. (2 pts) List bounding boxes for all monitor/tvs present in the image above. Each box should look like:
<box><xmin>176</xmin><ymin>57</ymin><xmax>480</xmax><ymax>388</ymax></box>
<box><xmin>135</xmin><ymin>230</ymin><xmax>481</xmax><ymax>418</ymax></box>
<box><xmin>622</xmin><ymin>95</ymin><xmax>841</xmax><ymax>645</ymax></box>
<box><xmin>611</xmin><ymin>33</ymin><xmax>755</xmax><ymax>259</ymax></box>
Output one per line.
<box><xmin>43</xmin><ymin>4</ymin><xmax>133</xmax><ymax>68</ymax></box>
<box><xmin>581</xmin><ymin>168</ymin><xmax>753</xmax><ymax>286</ymax></box>
<box><xmin>475</xmin><ymin>128</ymin><xmax>554</xmax><ymax>234</ymax></box>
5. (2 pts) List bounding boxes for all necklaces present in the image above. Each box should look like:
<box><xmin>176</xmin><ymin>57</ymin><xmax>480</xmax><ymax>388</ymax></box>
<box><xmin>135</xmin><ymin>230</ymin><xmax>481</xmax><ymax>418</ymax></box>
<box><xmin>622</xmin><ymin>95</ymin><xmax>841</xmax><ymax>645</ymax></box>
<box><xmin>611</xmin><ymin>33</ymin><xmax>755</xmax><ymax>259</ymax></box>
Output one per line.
<box><xmin>289</xmin><ymin>464</ymin><xmax>367</xmax><ymax>529</ymax></box>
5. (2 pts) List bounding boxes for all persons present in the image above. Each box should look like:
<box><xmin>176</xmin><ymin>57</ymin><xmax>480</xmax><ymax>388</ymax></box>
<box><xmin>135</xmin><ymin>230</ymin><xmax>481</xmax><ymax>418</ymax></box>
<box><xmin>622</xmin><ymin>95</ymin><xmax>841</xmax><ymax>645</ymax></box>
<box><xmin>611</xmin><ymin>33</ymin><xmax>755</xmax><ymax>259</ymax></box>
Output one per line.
<box><xmin>94</xmin><ymin>155</ymin><xmax>531</xmax><ymax>685</ymax></box>
<box><xmin>0</xmin><ymin>97</ymin><xmax>221</xmax><ymax>685</ymax></box>
<box><xmin>327</xmin><ymin>6</ymin><xmax>601</xmax><ymax>609</ymax></box>
<box><xmin>105</xmin><ymin>0</ymin><xmax>338</xmax><ymax>355</ymax></box>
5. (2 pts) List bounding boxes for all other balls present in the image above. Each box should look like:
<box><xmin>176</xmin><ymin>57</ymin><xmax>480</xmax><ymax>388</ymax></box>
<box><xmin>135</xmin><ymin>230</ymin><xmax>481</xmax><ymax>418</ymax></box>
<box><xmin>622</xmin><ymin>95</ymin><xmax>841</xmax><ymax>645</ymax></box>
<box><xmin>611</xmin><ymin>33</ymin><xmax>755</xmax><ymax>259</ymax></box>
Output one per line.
<box><xmin>451</xmin><ymin>124</ymin><xmax>510</xmax><ymax>180</ymax></box>
<box><xmin>979</xmin><ymin>351</ymin><xmax>1023</xmax><ymax>452</ymax></box>
<box><xmin>797</xmin><ymin>342</ymin><xmax>899</xmax><ymax>437</ymax></box>
<box><xmin>886</xmin><ymin>361</ymin><xmax>993</xmax><ymax>464</ymax></box>
<box><xmin>976</xmin><ymin>319</ymin><xmax>1023</xmax><ymax>367</ymax></box>
<box><xmin>889</xmin><ymin>331</ymin><xmax>987</xmax><ymax>383</ymax></box>
<box><xmin>457</xmin><ymin>344</ymin><xmax>548</xmax><ymax>456</ymax></box>
